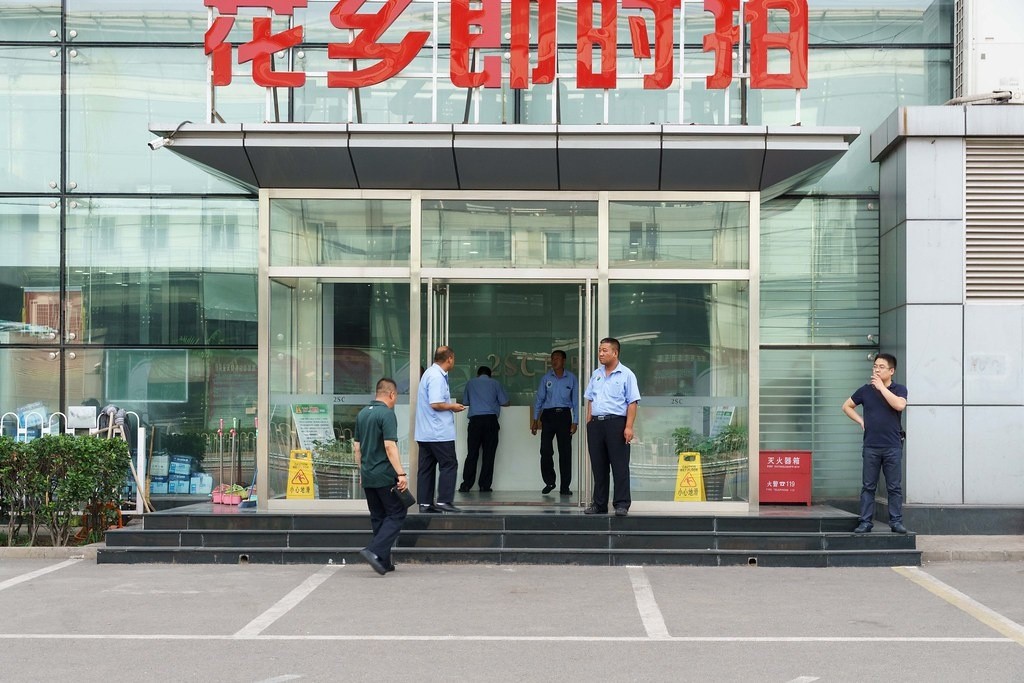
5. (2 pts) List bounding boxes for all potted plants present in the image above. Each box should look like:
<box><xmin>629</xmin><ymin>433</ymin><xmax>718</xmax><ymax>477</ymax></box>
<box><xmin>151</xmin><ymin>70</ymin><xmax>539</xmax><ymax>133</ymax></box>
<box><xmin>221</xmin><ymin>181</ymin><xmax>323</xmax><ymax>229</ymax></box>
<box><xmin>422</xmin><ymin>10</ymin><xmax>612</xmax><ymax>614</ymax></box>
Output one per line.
<box><xmin>671</xmin><ymin>427</ymin><xmax>749</xmax><ymax>502</ymax></box>
<box><xmin>310</xmin><ymin>427</ymin><xmax>366</xmax><ymax>501</ymax></box>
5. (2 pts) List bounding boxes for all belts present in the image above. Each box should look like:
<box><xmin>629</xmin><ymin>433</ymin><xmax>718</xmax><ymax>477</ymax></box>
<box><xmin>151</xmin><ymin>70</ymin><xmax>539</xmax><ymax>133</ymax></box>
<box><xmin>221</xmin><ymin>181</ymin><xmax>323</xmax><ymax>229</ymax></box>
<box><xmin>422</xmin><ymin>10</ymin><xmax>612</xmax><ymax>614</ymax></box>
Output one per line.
<box><xmin>593</xmin><ymin>414</ymin><xmax>619</xmax><ymax>421</ymax></box>
<box><xmin>544</xmin><ymin>406</ymin><xmax>570</xmax><ymax>412</ymax></box>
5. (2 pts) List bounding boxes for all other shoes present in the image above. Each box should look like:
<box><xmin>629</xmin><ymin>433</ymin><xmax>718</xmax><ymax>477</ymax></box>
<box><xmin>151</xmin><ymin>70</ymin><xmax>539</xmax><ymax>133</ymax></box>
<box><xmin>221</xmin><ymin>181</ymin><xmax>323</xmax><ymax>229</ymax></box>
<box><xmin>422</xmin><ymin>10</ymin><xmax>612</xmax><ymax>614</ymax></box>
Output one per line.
<box><xmin>561</xmin><ymin>489</ymin><xmax>572</xmax><ymax>495</ymax></box>
<box><xmin>542</xmin><ymin>485</ymin><xmax>556</xmax><ymax>494</ymax></box>
<box><xmin>479</xmin><ymin>487</ymin><xmax>493</xmax><ymax>492</ymax></box>
<box><xmin>459</xmin><ymin>486</ymin><xmax>469</xmax><ymax>492</ymax></box>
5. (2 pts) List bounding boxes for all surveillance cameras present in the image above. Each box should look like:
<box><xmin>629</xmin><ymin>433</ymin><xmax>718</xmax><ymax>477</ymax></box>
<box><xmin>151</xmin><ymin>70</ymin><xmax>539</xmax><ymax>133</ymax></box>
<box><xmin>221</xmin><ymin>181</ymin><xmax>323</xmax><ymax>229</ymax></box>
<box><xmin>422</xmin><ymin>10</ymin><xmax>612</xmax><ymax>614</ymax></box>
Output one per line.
<box><xmin>148</xmin><ymin>136</ymin><xmax>170</xmax><ymax>150</ymax></box>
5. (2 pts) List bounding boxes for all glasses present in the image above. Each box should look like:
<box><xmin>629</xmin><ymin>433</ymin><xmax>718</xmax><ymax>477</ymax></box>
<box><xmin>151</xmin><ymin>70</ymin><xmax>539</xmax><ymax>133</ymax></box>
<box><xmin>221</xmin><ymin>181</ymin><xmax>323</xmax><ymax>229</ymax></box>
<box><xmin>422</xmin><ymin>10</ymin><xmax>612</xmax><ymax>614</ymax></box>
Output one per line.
<box><xmin>872</xmin><ymin>365</ymin><xmax>891</xmax><ymax>371</ymax></box>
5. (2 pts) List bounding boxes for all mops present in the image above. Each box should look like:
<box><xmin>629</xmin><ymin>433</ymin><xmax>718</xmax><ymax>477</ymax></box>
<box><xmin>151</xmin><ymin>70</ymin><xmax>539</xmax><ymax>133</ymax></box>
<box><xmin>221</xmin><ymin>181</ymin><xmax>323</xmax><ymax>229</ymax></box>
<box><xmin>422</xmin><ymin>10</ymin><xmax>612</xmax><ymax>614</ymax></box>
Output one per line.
<box><xmin>101</xmin><ymin>405</ymin><xmax>150</xmax><ymax>513</ymax></box>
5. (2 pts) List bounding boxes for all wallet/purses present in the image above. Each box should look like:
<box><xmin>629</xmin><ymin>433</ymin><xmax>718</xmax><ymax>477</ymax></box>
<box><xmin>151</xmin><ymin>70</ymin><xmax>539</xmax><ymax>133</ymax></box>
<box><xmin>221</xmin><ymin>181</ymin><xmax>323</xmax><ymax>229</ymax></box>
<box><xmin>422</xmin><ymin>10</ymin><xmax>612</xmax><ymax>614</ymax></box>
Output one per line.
<box><xmin>390</xmin><ymin>484</ymin><xmax>416</xmax><ymax>508</ymax></box>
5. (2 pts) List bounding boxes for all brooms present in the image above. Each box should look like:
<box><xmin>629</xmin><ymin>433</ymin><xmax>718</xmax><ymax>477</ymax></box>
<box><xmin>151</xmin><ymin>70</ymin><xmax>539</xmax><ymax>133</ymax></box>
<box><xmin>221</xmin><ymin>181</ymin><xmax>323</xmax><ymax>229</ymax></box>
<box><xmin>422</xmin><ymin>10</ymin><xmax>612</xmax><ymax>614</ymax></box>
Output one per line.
<box><xmin>213</xmin><ymin>418</ymin><xmax>258</xmax><ymax>508</ymax></box>
<box><xmin>143</xmin><ymin>426</ymin><xmax>156</xmax><ymax>513</ymax></box>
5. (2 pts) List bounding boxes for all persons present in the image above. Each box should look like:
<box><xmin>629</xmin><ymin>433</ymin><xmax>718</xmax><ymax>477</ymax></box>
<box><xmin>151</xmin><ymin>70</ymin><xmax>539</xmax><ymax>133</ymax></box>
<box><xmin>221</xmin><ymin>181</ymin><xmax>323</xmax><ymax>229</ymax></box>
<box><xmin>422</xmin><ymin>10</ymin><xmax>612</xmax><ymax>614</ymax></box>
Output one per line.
<box><xmin>842</xmin><ymin>353</ymin><xmax>908</xmax><ymax>534</ymax></box>
<box><xmin>532</xmin><ymin>350</ymin><xmax>579</xmax><ymax>495</ymax></box>
<box><xmin>414</xmin><ymin>346</ymin><xmax>466</xmax><ymax>513</ymax></box>
<box><xmin>458</xmin><ymin>365</ymin><xmax>510</xmax><ymax>493</ymax></box>
<box><xmin>584</xmin><ymin>337</ymin><xmax>642</xmax><ymax>516</ymax></box>
<box><xmin>354</xmin><ymin>378</ymin><xmax>409</xmax><ymax>576</ymax></box>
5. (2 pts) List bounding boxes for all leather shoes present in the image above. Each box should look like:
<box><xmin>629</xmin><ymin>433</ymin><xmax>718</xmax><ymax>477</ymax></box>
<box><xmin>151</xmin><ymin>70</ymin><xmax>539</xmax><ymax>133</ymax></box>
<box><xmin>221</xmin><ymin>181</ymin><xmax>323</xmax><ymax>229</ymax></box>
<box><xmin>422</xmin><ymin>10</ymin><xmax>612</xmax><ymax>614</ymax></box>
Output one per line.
<box><xmin>419</xmin><ymin>503</ymin><xmax>442</xmax><ymax>513</ymax></box>
<box><xmin>388</xmin><ymin>565</ymin><xmax>395</xmax><ymax>570</ymax></box>
<box><xmin>360</xmin><ymin>548</ymin><xmax>386</xmax><ymax>575</ymax></box>
<box><xmin>855</xmin><ymin>523</ymin><xmax>872</xmax><ymax>532</ymax></box>
<box><xmin>436</xmin><ymin>502</ymin><xmax>460</xmax><ymax>512</ymax></box>
<box><xmin>891</xmin><ymin>524</ymin><xmax>907</xmax><ymax>533</ymax></box>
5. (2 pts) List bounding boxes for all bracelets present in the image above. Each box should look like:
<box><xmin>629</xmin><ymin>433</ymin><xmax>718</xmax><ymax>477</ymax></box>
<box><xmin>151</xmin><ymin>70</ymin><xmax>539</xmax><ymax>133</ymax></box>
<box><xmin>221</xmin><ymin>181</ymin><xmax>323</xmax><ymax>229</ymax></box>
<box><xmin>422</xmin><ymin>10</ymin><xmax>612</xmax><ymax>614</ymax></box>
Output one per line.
<box><xmin>396</xmin><ymin>473</ymin><xmax>407</xmax><ymax>476</ymax></box>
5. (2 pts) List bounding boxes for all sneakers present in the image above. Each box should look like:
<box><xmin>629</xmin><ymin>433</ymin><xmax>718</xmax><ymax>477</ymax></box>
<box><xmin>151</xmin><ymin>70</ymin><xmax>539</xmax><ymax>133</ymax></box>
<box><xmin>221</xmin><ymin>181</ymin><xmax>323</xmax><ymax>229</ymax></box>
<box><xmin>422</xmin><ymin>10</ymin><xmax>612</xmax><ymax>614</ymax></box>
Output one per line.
<box><xmin>616</xmin><ymin>507</ymin><xmax>627</xmax><ymax>516</ymax></box>
<box><xmin>585</xmin><ymin>503</ymin><xmax>608</xmax><ymax>514</ymax></box>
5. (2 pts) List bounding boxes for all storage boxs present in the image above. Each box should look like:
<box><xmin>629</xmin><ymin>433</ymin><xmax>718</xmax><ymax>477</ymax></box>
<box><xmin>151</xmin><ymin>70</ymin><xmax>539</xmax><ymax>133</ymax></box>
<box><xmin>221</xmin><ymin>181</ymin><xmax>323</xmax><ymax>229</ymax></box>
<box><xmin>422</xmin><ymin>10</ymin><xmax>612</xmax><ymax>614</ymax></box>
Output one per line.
<box><xmin>0</xmin><ymin>399</ymin><xmax>62</xmax><ymax>445</ymax></box>
<box><xmin>124</xmin><ymin>448</ymin><xmax>213</xmax><ymax>495</ymax></box>
